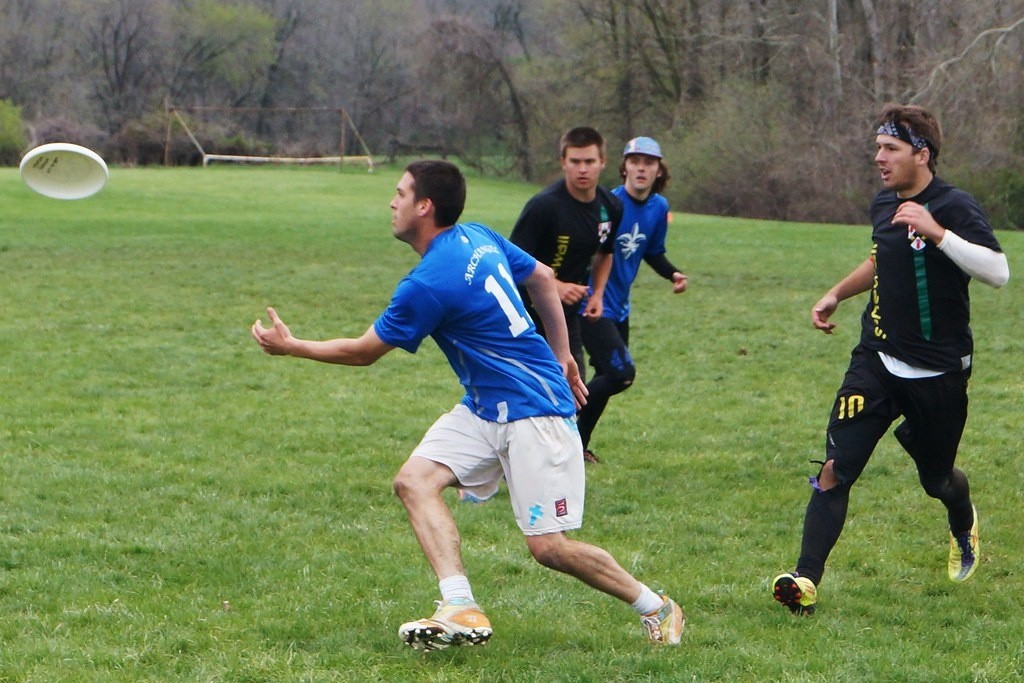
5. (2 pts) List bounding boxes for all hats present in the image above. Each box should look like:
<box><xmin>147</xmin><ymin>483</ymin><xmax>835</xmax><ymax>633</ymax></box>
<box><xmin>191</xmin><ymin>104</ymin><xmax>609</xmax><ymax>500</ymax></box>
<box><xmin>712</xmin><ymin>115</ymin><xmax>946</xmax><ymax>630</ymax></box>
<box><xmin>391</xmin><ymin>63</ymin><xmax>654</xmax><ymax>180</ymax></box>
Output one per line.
<box><xmin>623</xmin><ymin>136</ymin><xmax>663</xmax><ymax>159</ymax></box>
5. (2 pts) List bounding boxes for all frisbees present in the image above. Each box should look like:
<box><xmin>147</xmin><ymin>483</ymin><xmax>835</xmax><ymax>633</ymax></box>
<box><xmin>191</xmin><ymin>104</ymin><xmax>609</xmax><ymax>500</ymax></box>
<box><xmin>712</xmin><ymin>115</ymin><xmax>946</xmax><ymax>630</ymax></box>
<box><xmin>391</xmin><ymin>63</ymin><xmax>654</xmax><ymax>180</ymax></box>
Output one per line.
<box><xmin>20</xmin><ymin>143</ymin><xmax>110</xmax><ymax>200</ymax></box>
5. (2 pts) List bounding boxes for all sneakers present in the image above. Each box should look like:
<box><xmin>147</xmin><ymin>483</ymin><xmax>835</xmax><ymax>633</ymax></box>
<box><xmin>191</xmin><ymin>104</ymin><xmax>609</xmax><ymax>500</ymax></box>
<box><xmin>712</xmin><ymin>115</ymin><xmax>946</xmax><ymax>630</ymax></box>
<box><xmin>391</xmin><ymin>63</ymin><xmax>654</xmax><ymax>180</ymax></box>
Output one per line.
<box><xmin>772</xmin><ymin>574</ymin><xmax>819</xmax><ymax>620</ymax></box>
<box><xmin>584</xmin><ymin>450</ymin><xmax>600</xmax><ymax>465</ymax></box>
<box><xmin>457</xmin><ymin>488</ymin><xmax>488</xmax><ymax>506</ymax></box>
<box><xmin>947</xmin><ymin>503</ymin><xmax>981</xmax><ymax>585</ymax></box>
<box><xmin>397</xmin><ymin>603</ymin><xmax>494</xmax><ymax>654</ymax></box>
<box><xmin>640</xmin><ymin>593</ymin><xmax>686</xmax><ymax>647</ymax></box>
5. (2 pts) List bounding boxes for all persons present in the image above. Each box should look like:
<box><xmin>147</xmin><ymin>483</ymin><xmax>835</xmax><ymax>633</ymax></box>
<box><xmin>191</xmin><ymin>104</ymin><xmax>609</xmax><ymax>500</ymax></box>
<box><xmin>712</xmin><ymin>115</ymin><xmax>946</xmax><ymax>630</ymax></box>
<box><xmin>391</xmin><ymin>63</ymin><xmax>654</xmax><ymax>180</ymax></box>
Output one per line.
<box><xmin>507</xmin><ymin>125</ymin><xmax>624</xmax><ymax>423</ymax></box>
<box><xmin>773</xmin><ymin>102</ymin><xmax>1011</xmax><ymax>617</ymax></box>
<box><xmin>576</xmin><ymin>135</ymin><xmax>690</xmax><ymax>465</ymax></box>
<box><xmin>250</xmin><ymin>159</ymin><xmax>685</xmax><ymax>653</ymax></box>
